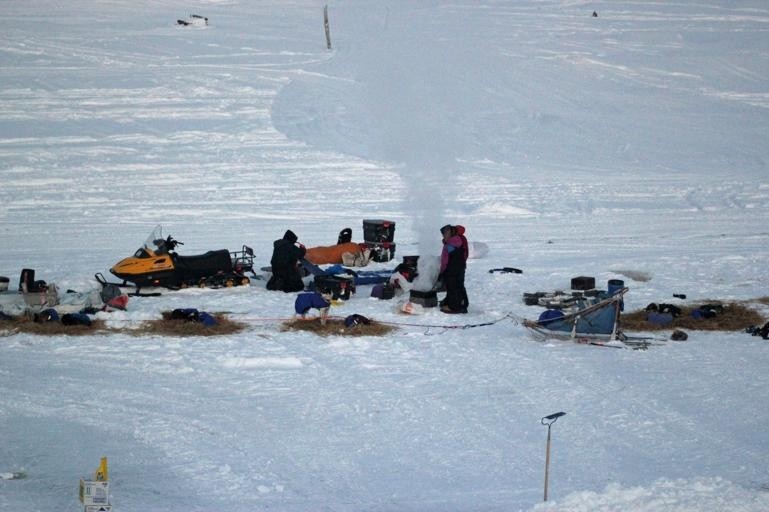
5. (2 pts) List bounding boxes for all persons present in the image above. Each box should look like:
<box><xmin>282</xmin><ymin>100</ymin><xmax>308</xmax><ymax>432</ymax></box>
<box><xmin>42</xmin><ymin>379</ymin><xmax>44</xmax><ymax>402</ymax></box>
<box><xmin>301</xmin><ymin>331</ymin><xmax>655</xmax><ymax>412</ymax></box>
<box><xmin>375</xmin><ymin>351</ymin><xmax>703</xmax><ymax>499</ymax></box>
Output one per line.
<box><xmin>437</xmin><ymin>225</ymin><xmax>469</xmax><ymax>314</ymax></box>
<box><xmin>269</xmin><ymin>229</ymin><xmax>318</xmax><ymax>278</ymax></box>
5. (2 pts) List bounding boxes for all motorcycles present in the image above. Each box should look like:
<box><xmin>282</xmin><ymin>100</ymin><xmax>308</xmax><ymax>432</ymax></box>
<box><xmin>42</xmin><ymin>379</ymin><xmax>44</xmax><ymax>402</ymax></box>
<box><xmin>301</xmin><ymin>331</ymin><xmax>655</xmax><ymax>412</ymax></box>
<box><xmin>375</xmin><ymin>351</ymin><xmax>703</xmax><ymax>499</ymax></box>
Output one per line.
<box><xmin>95</xmin><ymin>225</ymin><xmax>256</xmax><ymax>297</ymax></box>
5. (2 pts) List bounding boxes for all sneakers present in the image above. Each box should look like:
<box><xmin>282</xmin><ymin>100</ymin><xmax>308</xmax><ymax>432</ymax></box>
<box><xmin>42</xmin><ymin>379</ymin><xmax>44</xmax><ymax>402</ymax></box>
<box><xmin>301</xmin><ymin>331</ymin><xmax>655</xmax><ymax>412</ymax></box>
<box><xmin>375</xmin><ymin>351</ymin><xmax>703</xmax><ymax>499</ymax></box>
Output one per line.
<box><xmin>440</xmin><ymin>300</ymin><xmax>467</xmax><ymax>313</ymax></box>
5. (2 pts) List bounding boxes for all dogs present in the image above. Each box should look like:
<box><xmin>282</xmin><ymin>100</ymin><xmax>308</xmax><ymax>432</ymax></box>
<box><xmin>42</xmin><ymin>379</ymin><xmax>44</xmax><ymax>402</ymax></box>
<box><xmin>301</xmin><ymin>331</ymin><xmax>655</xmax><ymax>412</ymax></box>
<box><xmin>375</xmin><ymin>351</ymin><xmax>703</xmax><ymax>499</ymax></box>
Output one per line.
<box><xmin>291</xmin><ymin>286</ymin><xmax>334</xmax><ymax>327</ymax></box>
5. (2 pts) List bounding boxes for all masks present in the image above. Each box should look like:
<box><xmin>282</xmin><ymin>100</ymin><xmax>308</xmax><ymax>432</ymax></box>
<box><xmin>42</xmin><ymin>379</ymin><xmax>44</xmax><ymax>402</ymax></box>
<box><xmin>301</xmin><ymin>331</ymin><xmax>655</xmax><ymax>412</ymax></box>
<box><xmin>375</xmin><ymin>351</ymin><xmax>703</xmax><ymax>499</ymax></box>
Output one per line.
<box><xmin>448</xmin><ymin>245</ymin><xmax>454</xmax><ymax>252</ymax></box>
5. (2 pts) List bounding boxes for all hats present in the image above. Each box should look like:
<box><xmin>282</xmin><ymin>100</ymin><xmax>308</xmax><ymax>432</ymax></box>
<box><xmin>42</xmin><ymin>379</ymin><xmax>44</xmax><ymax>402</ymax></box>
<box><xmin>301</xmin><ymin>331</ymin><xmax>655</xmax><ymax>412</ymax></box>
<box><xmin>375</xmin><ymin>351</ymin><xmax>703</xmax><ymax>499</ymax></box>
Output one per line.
<box><xmin>448</xmin><ymin>236</ymin><xmax>462</xmax><ymax>247</ymax></box>
